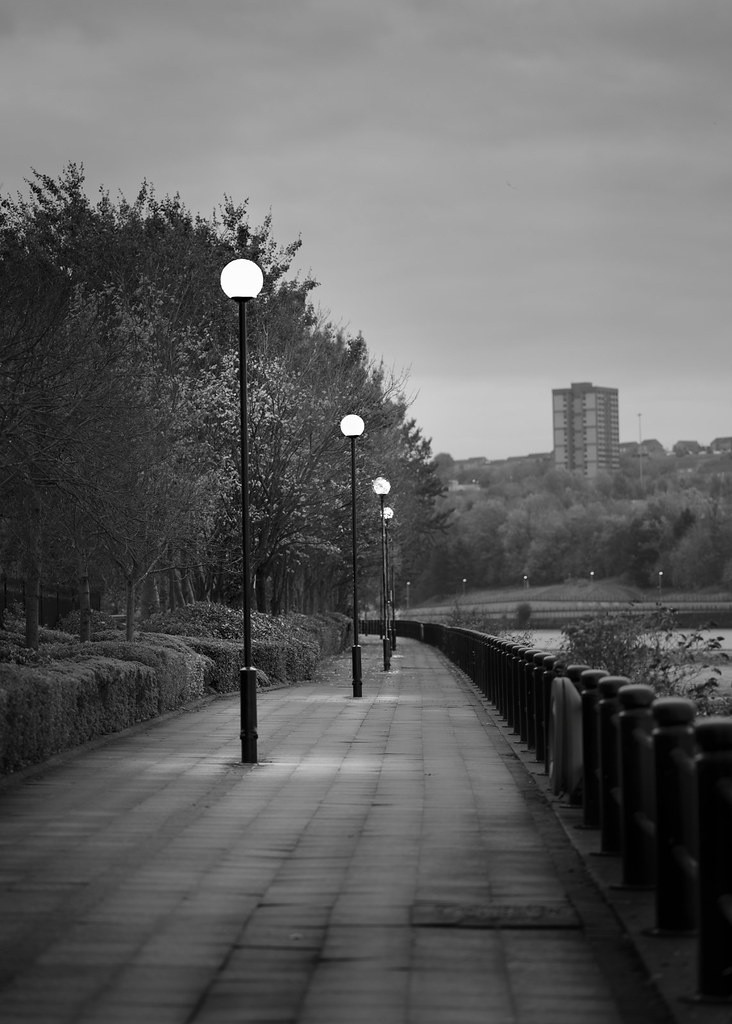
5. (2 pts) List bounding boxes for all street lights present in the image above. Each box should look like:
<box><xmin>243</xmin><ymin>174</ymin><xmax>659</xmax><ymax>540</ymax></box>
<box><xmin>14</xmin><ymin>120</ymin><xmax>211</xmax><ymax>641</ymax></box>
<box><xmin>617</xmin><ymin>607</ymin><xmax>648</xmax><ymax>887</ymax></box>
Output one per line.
<box><xmin>462</xmin><ymin>578</ymin><xmax>468</xmax><ymax>598</ymax></box>
<box><xmin>217</xmin><ymin>254</ymin><xmax>267</xmax><ymax>762</ymax></box>
<box><xmin>339</xmin><ymin>413</ymin><xmax>365</xmax><ymax>698</ymax></box>
<box><xmin>522</xmin><ymin>576</ymin><xmax>528</xmax><ymax>595</ymax></box>
<box><xmin>589</xmin><ymin>571</ymin><xmax>595</xmax><ymax>597</ymax></box>
<box><xmin>406</xmin><ymin>580</ymin><xmax>411</xmax><ymax>608</ymax></box>
<box><xmin>657</xmin><ymin>571</ymin><xmax>664</xmax><ymax>599</ymax></box>
<box><xmin>380</xmin><ymin>507</ymin><xmax>394</xmax><ymax>658</ymax></box>
<box><xmin>371</xmin><ymin>476</ymin><xmax>392</xmax><ymax>671</ymax></box>
